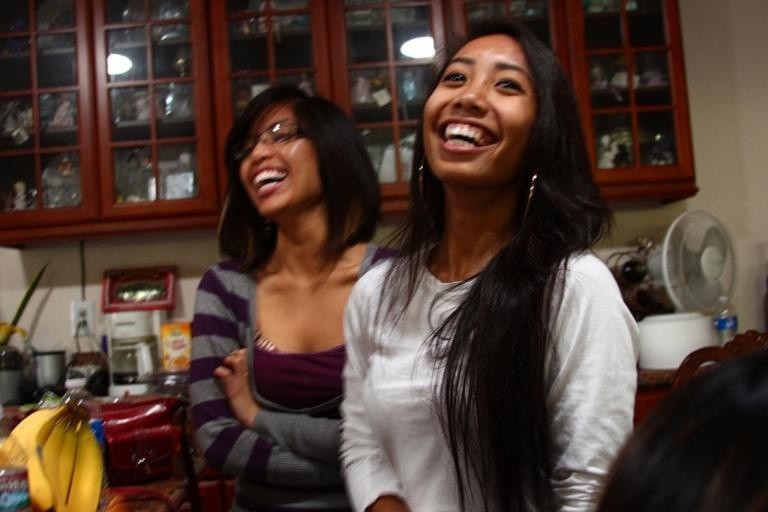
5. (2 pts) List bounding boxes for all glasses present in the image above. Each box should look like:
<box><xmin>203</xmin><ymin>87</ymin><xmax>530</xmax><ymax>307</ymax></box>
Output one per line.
<box><xmin>231</xmin><ymin>117</ymin><xmax>317</xmax><ymax>166</ymax></box>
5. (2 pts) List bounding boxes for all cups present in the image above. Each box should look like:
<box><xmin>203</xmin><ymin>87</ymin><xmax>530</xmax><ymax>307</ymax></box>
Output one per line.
<box><xmin>30</xmin><ymin>350</ymin><xmax>68</xmax><ymax>391</ymax></box>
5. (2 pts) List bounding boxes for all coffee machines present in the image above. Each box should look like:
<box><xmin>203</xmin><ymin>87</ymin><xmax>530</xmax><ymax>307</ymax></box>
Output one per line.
<box><xmin>105</xmin><ymin>312</ymin><xmax>161</xmax><ymax>399</ymax></box>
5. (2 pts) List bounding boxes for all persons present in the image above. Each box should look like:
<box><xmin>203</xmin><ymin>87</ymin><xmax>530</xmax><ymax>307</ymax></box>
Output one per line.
<box><xmin>338</xmin><ymin>24</ymin><xmax>641</xmax><ymax>512</ymax></box>
<box><xmin>190</xmin><ymin>81</ymin><xmax>401</xmax><ymax>512</ymax></box>
<box><xmin>597</xmin><ymin>351</ymin><xmax>768</xmax><ymax>512</ymax></box>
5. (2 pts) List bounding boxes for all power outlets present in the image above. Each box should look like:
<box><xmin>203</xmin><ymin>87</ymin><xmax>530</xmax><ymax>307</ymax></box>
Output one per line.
<box><xmin>69</xmin><ymin>299</ymin><xmax>94</xmax><ymax>339</ymax></box>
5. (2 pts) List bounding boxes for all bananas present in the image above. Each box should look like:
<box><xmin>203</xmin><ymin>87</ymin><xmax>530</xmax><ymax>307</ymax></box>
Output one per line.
<box><xmin>7</xmin><ymin>396</ymin><xmax>105</xmax><ymax>511</ymax></box>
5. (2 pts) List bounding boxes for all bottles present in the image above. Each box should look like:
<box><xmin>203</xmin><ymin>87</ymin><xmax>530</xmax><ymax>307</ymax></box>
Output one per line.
<box><xmin>61</xmin><ymin>377</ymin><xmax>111</xmax><ymax>510</ymax></box>
<box><xmin>0</xmin><ymin>346</ymin><xmax>23</xmax><ymax>404</ymax></box>
<box><xmin>1</xmin><ymin>409</ymin><xmax>33</xmax><ymax>511</ymax></box>
<box><xmin>712</xmin><ymin>294</ymin><xmax>738</xmax><ymax>349</ymax></box>
<box><xmin>0</xmin><ymin>0</ymin><xmax>673</xmax><ymax>207</ymax></box>
<box><xmin>159</xmin><ymin>368</ymin><xmax>189</xmax><ymax>395</ymax></box>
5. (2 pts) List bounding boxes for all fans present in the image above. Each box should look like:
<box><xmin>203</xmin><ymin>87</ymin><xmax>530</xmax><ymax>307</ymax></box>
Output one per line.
<box><xmin>660</xmin><ymin>202</ymin><xmax>740</xmax><ymax>319</ymax></box>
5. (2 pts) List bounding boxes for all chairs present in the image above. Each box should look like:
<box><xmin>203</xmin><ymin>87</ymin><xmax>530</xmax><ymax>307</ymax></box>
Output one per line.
<box><xmin>672</xmin><ymin>327</ymin><xmax>766</xmax><ymax>393</ymax></box>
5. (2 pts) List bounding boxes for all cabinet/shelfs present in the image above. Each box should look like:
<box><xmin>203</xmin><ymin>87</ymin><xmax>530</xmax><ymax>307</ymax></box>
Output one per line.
<box><xmin>445</xmin><ymin>1</ymin><xmax>701</xmax><ymax>208</ymax></box>
<box><xmin>0</xmin><ymin>1</ymin><xmax>218</xmax><ymax>250</ymax></box>
<box><xmin>209</xmin><ymin>0</ymin><xmax>457</xmax><ymax>220</ymax></box>
<box><xmin>1</xmin><ymin>356</ymin><xmax>681</xmax><ymax>510</ymax></box>
